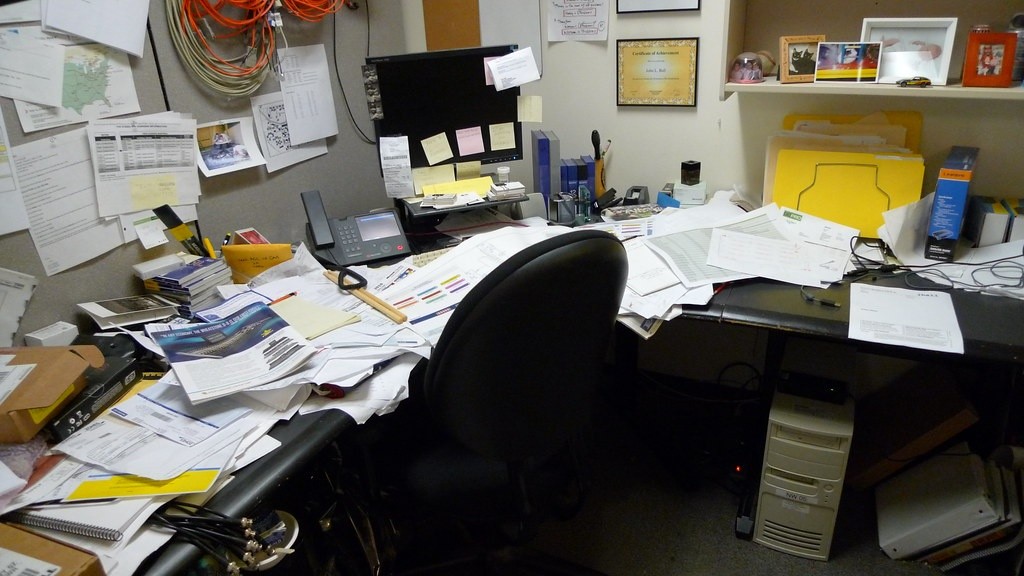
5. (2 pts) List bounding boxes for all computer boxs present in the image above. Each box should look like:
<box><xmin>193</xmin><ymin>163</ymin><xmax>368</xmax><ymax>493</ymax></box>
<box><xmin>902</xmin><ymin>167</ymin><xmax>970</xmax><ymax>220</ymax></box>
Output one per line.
<box><xmin>752</xmin><ymin>391</ymin><xmax>855</xmax><ymax>561</ymax></box>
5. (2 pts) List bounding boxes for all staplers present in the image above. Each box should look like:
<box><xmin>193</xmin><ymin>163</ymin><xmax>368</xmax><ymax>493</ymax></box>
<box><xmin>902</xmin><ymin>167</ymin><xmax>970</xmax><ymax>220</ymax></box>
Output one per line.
<box><xmin>591</xmin><ymin>188</ymin><xmax>622</xmax><ymax>212</ymax></box>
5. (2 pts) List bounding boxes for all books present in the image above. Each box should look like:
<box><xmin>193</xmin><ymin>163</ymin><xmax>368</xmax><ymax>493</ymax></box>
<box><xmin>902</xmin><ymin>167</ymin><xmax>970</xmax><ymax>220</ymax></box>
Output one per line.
<box><xmin>270</xmin><ymin>295</ymin><xmax>361</xmax><ymax>340</ymax></box>
<box><xmin>144</xmin><ymin>251</ymin><xmax>233</xmax><ymax>319</ymax></box>
<box><xmin>77</xmin><ymin>294</ymin><xmax>181</xmax><ymax>329</ymax></box>
<box><xmin>1</xmin><ymin>495</ymin><xmax>162</xmax><ymax>542</ymax></box>
<box><xmin>875</xmin><ymin>445</ymin><xmax>1020</xmax><ymax>565</ymax></box>
<box><xmin>152</xmin><ymin>301</ymin><xmax>318</xmax><ymax>406</ymax></box>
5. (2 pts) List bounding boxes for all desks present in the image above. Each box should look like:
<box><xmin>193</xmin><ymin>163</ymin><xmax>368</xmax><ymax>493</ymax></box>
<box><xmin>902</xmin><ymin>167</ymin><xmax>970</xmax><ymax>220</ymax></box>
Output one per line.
<box><xmin>136</xmin><ymin>234</ymin><xmax>1024</xmax><ymax>576</ymax></box>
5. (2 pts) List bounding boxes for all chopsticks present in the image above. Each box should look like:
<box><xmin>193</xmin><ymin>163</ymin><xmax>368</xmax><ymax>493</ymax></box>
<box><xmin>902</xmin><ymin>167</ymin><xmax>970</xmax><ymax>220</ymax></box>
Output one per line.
<box><xmin>325</xmin><ymin>270</ymin><xmax>407</xmax><ymax>325</ymax></box>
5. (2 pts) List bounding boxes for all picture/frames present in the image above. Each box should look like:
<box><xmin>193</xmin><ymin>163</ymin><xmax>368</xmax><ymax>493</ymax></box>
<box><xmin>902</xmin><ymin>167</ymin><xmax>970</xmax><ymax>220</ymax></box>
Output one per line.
<box><xmin>963</xmin><ymin>30</ymin><xmax>1018</xmax><ymax>87</ymax></box>
<box><xmin>778</xmin><ymin>34</ymin><xmax>834</xmax><ymax>81</ymax></box>
<box><xmin>858</xmin><ymin>17</ymin><xmax>961</xmax><ymax>86</ymax></box>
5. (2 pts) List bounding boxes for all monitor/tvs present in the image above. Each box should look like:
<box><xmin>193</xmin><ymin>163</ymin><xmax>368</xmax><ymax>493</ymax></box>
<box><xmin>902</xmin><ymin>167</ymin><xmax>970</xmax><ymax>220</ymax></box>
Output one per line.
<box><xmin>365</xmin><ymin>43</ymin><xmax>523</xmax><ymax>177</ymax></box>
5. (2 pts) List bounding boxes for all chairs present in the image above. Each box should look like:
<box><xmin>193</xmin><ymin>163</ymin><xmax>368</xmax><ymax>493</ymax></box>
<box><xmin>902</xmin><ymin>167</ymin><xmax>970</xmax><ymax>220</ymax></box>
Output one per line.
<box><xmin>385</xmin><ymin>229</ymin><xmax>627</xmax><ymax>553</ymax></box>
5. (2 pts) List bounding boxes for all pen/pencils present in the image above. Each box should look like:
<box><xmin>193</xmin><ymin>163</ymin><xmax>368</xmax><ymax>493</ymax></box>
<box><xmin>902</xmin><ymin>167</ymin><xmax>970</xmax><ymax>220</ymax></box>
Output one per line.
<box><xmin>204</xmin><ymin>238</ymin><xmax>216</xmax><ymax>259</ymax></box>
<box><xmin>715</xmin><ymin>284</ymin><xmax>726</xmax><ymax>295</ymax></box>
<box><xmin>267</xmin><ymin>292</ymin><xmax>297</xmax><ymax>306</ymax></box>
<box><xmin>220</xmin><ymin>233</ymin><xmax>231</xmax><ymax>251</ymax></box>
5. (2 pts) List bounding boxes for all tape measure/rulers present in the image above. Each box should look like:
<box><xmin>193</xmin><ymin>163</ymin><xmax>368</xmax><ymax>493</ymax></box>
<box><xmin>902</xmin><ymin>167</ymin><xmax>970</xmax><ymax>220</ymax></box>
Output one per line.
<box><xmin>152</xmin><ymin>204</ymin><xmax>210</xmax><ymax>257</ymax></box>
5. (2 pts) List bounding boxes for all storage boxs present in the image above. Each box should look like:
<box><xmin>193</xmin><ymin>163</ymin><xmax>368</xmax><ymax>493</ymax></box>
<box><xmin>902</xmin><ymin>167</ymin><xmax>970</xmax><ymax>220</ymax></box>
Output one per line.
<box><xmin>0</xmin><ymin>346</ymin><xmax>108</xmax><ymax>442</ymax></box>
<box><xmin>926</xmin><ymin>145</ymin><xmax>1024</xmax><ymax>263</ymax></box>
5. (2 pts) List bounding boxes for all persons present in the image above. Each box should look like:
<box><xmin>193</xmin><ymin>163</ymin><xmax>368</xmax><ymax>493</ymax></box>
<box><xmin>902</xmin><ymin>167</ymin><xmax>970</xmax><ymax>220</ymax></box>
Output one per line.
<box><xmin>732</xmin><ymin>62</ymin><xmax>761</xmax><ymax>80</ymax></box>
<box><xmin>214</xmin><ymin>134</ymin><xmax>223</xmax><ymax>150</ymax></box>
<box><xmin>881</xmin><ymin>35</ymin><xmax>941</xmax><ymax>77</ymax></box>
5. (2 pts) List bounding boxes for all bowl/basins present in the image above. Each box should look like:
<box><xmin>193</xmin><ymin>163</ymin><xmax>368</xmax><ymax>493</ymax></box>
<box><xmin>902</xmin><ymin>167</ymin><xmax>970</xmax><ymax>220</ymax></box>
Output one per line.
<box><xmin>229</xmin><ymin>509</ymin><xmax>301</xmax><ymax>572</ymax></box>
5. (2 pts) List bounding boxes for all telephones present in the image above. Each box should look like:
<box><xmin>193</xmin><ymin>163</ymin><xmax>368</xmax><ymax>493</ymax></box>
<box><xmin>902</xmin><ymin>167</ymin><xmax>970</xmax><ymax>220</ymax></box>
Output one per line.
<box><xmin>300</xmin><ymin>189</ymin><xmax>411</xmax><ymax>270</ymax></box>
